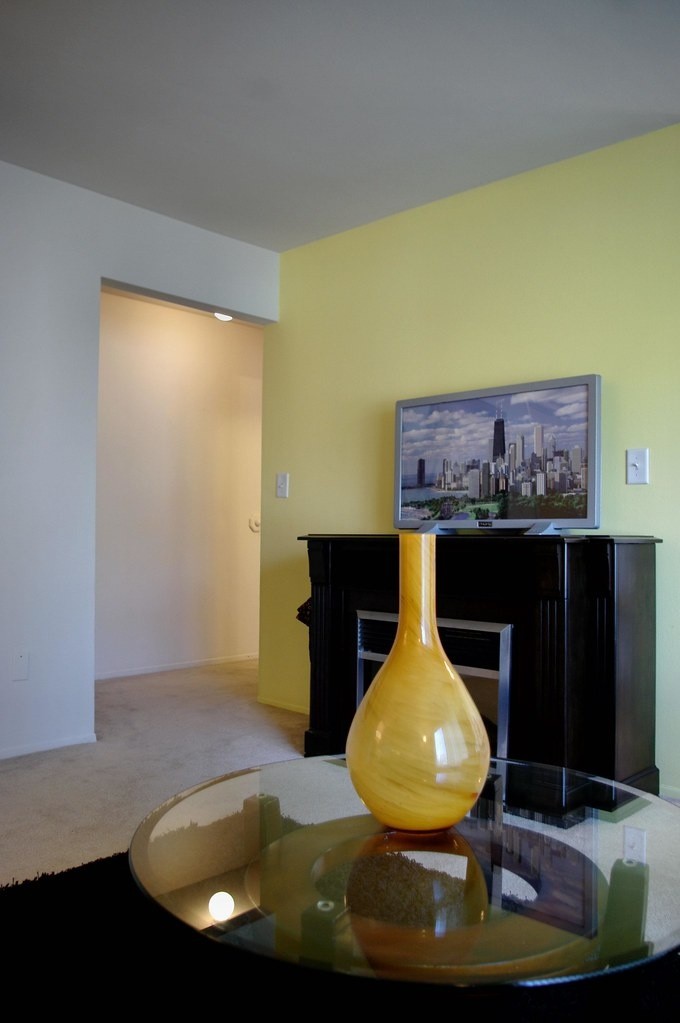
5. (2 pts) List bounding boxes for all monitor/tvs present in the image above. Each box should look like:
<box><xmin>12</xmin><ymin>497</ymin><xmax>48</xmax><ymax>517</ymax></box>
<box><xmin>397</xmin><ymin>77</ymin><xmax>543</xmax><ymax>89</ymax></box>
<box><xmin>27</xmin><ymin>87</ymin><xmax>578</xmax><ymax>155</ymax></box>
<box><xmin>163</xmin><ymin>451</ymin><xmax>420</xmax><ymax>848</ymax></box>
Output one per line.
<box><xmin>393</xmin><ymin>373</ymin><xmax>602</xmax><ymax>532</ymax></box>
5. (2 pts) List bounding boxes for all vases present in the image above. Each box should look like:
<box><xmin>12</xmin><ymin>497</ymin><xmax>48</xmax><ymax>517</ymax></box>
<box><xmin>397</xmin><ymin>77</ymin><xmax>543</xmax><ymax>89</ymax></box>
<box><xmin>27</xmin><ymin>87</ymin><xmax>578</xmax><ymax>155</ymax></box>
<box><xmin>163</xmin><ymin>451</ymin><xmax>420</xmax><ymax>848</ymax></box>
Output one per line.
<box><xmin>345</xmin><ymin>535</ymin><xmax>491</xmax><ymax>832</ymax></box>
<box><xmin>348</xmin><ymin>826</ymin><xmax>492</xmax><ymax>983</ymax></box>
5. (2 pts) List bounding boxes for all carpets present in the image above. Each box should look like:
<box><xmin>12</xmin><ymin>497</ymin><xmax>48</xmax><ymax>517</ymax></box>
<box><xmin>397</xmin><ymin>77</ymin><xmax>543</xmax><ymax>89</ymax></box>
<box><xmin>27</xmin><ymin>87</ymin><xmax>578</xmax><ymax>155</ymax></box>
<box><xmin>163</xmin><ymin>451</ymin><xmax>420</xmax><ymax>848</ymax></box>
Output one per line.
<box><xmin>0</xmin><ymin>848</ymin><xmax>680</xmax><ymax>1023</ymax></box>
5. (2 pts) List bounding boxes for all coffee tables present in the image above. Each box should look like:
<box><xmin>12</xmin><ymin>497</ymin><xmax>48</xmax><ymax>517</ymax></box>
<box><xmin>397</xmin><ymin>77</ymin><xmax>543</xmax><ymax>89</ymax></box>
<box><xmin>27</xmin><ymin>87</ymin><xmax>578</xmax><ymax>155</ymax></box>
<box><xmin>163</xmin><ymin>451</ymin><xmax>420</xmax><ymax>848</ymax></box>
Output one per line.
<box><xmin>126</xmin><ymin>752</ymin><xmax>680</xmax><ymax>984</ymax></box>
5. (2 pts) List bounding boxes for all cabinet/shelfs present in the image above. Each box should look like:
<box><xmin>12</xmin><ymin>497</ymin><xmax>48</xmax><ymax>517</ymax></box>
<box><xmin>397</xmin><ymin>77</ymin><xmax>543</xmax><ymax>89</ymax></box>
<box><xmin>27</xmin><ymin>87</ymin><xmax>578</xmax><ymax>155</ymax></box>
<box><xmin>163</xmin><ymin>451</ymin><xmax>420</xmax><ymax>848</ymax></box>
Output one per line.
<box><xmin>297</xmin><ymin>532</ymin><xmax>664</xmax><ymax>829</ymax></box>
